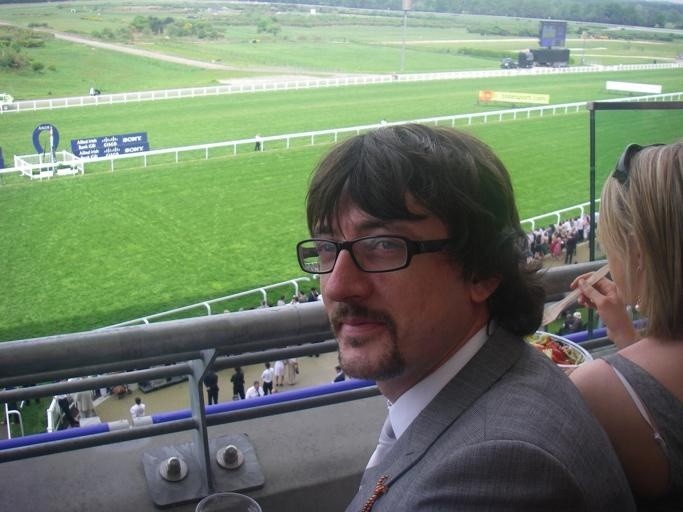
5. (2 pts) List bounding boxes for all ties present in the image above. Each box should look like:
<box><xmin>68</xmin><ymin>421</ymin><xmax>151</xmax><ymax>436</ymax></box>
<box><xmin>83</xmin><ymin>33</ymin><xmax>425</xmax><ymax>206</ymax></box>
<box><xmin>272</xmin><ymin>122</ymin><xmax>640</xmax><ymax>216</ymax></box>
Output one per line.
<box><xmin>359</xmin><ymin>412</ymin><xmax>397</xmax><ymax>491</ymax></box>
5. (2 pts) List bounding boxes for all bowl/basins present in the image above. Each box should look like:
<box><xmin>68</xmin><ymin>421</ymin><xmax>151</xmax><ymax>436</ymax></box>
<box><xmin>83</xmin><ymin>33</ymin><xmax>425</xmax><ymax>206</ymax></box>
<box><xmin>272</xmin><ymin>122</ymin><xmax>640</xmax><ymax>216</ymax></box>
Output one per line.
<box><xmin>524</xmin><ymin>330</ymin><xmax>593</xmax><ymax>377</ymax></box>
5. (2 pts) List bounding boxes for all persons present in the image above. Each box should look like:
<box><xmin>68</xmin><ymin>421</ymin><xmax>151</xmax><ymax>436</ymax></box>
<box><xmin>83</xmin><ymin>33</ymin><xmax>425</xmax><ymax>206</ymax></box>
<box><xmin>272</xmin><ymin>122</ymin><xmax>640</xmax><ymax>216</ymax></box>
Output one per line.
<box><xmin>304</xmin><ymin>123</ymin><xmax>637</xmax><ymax>512</ymax></box>
<box><xmin>254</xmin><ymin>133</ymin><xmax>261</xmax><ymax>152</ymax></box>
<box><xmin>568</xmin><ymin>143</ymin><xmax>682</xmax><ymax>512</ymax></box>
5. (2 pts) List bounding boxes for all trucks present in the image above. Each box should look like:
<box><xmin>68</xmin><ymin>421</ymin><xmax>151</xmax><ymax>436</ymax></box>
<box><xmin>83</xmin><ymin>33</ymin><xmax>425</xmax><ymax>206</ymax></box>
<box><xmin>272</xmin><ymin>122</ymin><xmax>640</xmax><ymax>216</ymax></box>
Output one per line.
<box><xmin>518</xmin><ymin>47</ymin><xmax>568</xmax><ymax>67</ymax></box>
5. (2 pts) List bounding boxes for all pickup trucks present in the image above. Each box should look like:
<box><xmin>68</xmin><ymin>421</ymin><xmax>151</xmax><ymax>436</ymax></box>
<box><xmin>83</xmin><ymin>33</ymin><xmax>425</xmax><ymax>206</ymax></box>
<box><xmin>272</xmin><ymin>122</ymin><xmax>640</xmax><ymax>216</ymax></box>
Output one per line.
<box><xmin>499</xmin><ymin>57</ymin><xmax>517</xmax><ymax>70</ymax></box>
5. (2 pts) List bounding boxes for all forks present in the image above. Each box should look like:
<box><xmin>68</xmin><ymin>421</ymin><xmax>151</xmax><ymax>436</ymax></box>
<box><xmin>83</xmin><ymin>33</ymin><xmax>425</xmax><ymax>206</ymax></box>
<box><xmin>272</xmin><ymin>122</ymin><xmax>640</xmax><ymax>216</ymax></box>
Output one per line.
<box><xmin>540</xmin><ymin>262</ymin><xmax>612</xmax><ymax>327</ymax></box>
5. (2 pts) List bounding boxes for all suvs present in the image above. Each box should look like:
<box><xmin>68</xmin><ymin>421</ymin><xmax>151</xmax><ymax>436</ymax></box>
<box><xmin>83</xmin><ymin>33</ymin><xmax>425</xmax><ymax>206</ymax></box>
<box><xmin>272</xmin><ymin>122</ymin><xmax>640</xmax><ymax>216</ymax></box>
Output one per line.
<box><xmin>0</xmin><ymin>93</ymin><xmax>14</xmax><ymax>111</ymax></box>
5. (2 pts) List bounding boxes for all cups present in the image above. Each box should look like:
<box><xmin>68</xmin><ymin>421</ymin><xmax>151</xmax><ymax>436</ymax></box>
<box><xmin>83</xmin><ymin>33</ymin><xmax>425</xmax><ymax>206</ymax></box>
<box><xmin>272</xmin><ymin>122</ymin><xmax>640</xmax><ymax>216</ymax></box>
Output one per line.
<box><xmin>195</xmin><ymin>491</ymin><xmax>262</xmax><ymax>512</ymax></box>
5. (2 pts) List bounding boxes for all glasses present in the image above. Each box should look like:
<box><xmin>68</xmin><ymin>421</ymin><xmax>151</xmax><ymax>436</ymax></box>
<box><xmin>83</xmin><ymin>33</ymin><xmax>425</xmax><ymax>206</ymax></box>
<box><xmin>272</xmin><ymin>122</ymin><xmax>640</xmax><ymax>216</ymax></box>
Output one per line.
<box><xmin>295</xmin><ymin>234</ymin><xmax>451</xmax><ymax>274</ymax></box>
<box><xmin>611</xmin><ymin>143</ymin><xmax>669</xmax><ymax>186</ymax></box>
